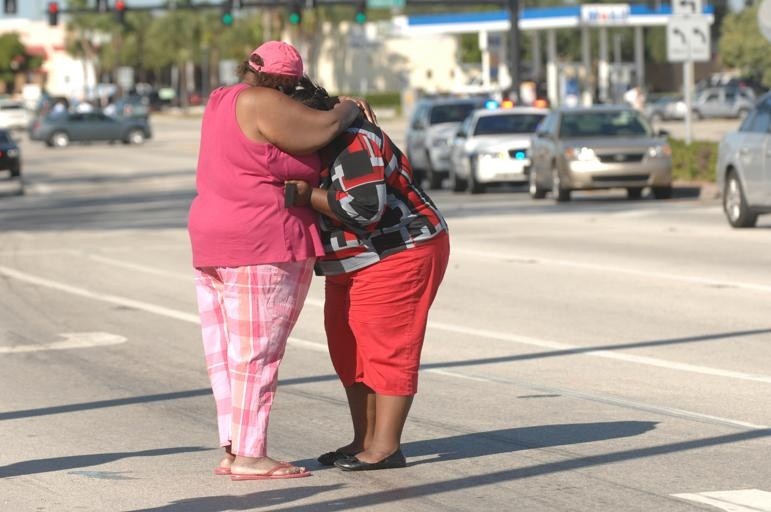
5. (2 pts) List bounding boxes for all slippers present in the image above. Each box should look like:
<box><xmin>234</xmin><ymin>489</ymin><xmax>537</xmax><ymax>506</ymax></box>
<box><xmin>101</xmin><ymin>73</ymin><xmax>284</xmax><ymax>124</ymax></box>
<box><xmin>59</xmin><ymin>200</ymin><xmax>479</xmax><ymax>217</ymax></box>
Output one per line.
<box><xmin>214</xmin><ymin>460</ymin><xmax>310</xmax><ymax>480</ymax></box>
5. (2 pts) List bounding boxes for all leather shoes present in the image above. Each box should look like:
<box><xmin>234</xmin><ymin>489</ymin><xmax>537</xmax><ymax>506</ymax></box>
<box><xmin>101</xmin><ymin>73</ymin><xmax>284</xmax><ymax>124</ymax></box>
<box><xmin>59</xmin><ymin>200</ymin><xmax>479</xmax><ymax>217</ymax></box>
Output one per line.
<box><xmin>334</xmin><ymin>450</ymin><xmax>405</xmax><ymax>471</ymax></box>
<box><xmin>317</xmin><ymin>452</ymin><xmax>359</xmax><ymax>466</ymax></box>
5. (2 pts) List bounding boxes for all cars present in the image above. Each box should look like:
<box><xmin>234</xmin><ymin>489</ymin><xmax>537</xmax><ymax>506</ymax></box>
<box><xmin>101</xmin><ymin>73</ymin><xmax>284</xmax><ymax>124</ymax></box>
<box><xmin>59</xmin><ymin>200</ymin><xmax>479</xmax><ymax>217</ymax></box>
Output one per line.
<box><xmin>27</xmin><ymin>110</ymin><xmax>152</xmax><ymax>149</ymax></box>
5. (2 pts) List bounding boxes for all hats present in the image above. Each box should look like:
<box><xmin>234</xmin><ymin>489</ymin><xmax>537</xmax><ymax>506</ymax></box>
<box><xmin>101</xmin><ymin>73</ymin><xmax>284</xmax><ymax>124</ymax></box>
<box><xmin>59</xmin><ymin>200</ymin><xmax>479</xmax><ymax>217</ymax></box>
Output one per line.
<box><xmin>248</xmin><ymin>41</ymin><xmax>303</xmax><ymax>80</ymax></box>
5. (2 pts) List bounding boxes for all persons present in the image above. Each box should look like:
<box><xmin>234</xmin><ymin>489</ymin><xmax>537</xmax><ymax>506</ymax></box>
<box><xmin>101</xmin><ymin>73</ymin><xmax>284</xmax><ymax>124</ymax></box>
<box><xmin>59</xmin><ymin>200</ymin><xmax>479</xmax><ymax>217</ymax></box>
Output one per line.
<box><xmin>190</xmin><ymin>38</ymin><xmax>374</xmax><ymax>478</ymax></box>
<box><xmin>277</xmin><ymin>82</ymin><xmax>451</xmax><ymax>470</ymax></box>
